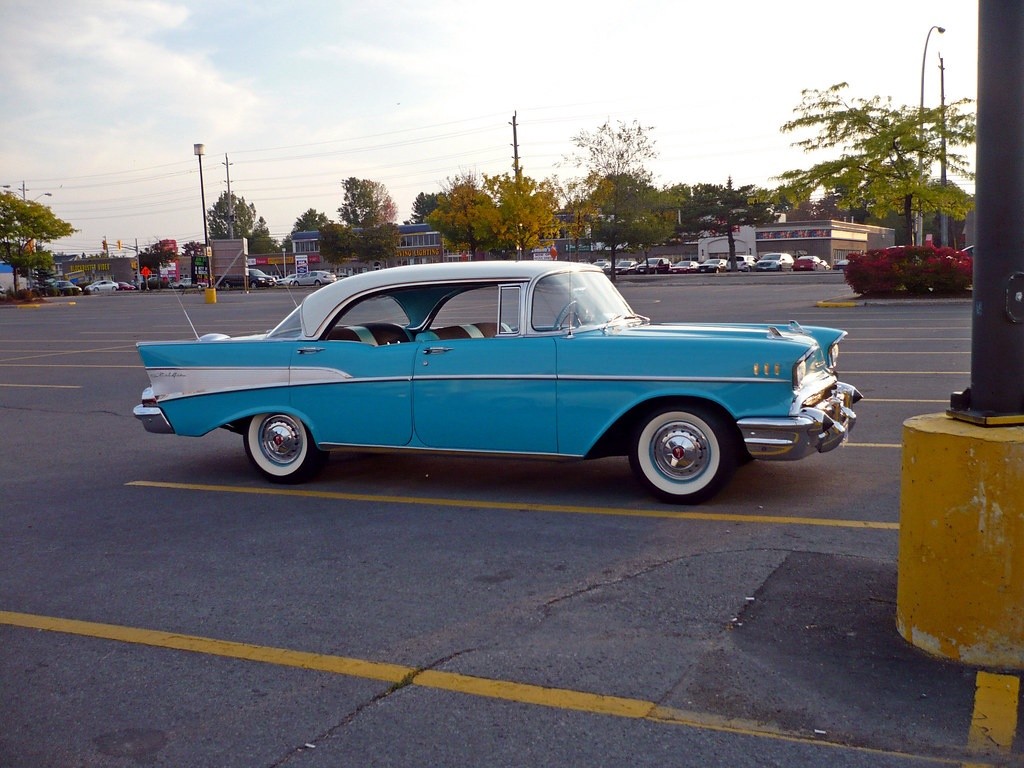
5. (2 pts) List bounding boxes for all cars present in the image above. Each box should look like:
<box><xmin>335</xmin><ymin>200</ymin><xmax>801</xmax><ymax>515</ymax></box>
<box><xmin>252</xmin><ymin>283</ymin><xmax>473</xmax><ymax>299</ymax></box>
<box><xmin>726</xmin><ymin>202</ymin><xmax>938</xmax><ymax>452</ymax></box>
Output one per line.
<box><xmin>44</xmin><ymin>281</ymin><xmax>83</xmax><ymax>297</ymax></box>
<box><xmin>85</xmin><ymin>280</ymin><xmax>120</xmax><ymax>293</ymax></box>
<box><xmin>276</xmin><ymin>274</ymin><xmax>296</xmax><ymax>286</ymax></box>
<box><xmin>615</xmin><ymin>260</ymin><xmax>640</xmax><ymax>275</ymax></box>
<box><xmin>133</xmin><ymin>258</ymin><xmax>865</xmax><ymax>508</ymax></box>
<box><xmin>667</xmin><ymin>260</ymin><xmax>700</xmax><ymax>274</ymax></box>
<box><xmin>592</xmin><ymin>261</ymin><xmax>612</xmax><ymax>275</ymax></box>
<box><xmin>168</xmin><ymin>277</ymin><xmax>206</xmax><ymax>289</ymax></box>
<box><xmin>961</xmin><ymin>244</ymin><xmax>974</xmax><ymax>259</ymax></box>
<box><xmin>118</xmin><ymin>282</ymin><xmax>136</xmax><ymax>290</ymax></box>
<box><xmin>755</xmin><ymin>253</ymin><xmax>795</xmax><ymax>271</ymax></box>
<box><xmin>725</xmin><ymin>254</ymin><xmax>760</xmax><ymax>272</ymax></box>
<box><xmin>698</xmin><ymin>258</ymin><xmax>728</xmax><ymax>273</ymax></box>
<box><xmin>291</xmin><ymin>270</ymin><xmax>338</xmax><ymax>286</ymax></box>
<box><xmin>793</xmin><ymin>255</ymin><xmax>821</xmax><ymax>271</ymax></box>
<box><xmin>141</xmin><ymin>277</ymin><xmax>168</xmax><ymax>289</ymax></box>
<box><xmin>635</xmin><ymin>258</ymin><xmax>672</xmax><ymax>275</ymax></box>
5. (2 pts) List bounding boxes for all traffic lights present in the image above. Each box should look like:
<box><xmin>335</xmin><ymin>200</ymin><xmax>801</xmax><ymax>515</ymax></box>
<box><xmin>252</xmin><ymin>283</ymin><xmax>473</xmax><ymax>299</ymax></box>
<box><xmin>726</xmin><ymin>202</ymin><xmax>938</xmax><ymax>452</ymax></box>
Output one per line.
<box><xmin>102</xmin><ymin>241</ymin><xmax>106</xmax><ymax>250</ymax></box>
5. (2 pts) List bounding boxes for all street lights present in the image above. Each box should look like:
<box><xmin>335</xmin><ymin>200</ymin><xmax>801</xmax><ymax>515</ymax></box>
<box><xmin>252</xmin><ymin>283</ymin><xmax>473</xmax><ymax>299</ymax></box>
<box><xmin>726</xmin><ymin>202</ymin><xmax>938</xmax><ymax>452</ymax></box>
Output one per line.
<box><xmin>915</xmin><ymin>24</ymin><xmax>946</xmax><ymax>246</ymax></box>
<box><xmin>193</xmin><ymin>143</ymin><xmax>217</xmax><ymax>304</ymax></box>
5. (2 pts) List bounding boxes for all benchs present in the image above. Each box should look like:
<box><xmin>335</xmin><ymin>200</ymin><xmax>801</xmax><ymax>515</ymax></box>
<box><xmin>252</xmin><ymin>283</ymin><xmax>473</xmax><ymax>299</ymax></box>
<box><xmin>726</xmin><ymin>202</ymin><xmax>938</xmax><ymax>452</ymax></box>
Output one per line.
<box><xmin>415</xmin><ymin>322</ymin><xmax>513</xmax><ymax>342</ymax></box>
<box><xmin>328</xmin><ymin>323</ymin><xmax>414</xmax><ymax>347</ymax></box>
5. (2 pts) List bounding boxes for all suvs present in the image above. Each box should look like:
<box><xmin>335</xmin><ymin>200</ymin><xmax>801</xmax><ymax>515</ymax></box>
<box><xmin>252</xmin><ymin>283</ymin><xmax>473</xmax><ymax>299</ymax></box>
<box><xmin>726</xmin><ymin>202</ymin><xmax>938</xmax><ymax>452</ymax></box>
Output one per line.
<box><xmin>224</xmin><ymin>269</ymin><xmax>277</xmax><ymax>289</ymax></box>
